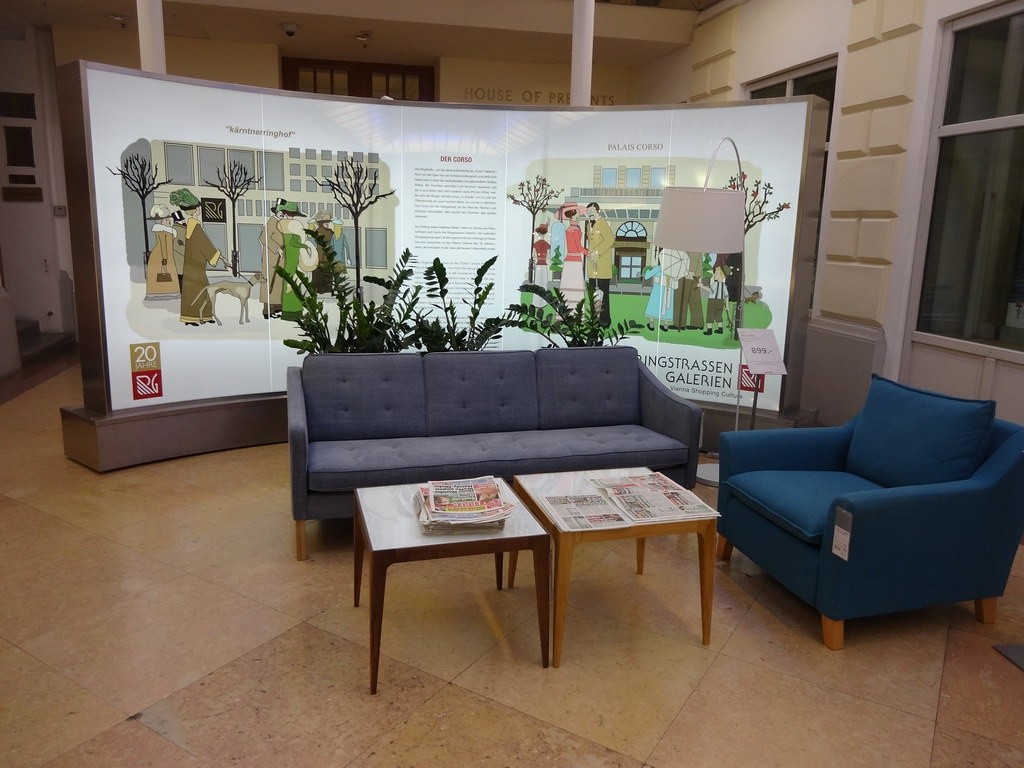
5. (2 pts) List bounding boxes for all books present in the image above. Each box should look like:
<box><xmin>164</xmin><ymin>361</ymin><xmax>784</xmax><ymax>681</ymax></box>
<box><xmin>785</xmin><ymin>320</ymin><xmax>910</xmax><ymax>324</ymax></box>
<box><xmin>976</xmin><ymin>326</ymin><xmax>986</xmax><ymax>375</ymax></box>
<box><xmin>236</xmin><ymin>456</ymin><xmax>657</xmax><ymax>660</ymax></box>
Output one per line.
<box><xmin>411</xmin><ymin>475</ymin><xmax>520</xmax><ymax>536</ymax></box>
<box><xmin>538</xmin><ymin>472</ymin><xmax>724</xmax><ymax>532</ymax></box>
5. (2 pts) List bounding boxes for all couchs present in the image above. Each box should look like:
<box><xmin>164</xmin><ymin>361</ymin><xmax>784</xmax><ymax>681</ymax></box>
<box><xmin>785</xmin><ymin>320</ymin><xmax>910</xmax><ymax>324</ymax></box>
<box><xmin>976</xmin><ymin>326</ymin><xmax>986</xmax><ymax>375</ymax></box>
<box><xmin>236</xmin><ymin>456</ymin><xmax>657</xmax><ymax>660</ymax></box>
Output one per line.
<box><xmin>717</xmin><ymin>418</ymin><xmax>1024</xmax><ymax>651</ymax></box>
<box><xmin>287</xmin><ymin>345</ymin><xmax>703</xmax><ymax>560</ymax></box>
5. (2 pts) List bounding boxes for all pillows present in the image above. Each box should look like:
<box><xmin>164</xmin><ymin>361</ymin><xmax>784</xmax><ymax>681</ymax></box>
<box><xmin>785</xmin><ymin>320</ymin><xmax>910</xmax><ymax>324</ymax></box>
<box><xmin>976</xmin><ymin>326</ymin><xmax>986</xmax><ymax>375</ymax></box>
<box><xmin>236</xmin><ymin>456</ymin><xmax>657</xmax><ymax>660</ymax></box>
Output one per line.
<box><xmin>844</xmin><ymin>372</ymin><xmax>997</xmax><ymax>489</ymax></box>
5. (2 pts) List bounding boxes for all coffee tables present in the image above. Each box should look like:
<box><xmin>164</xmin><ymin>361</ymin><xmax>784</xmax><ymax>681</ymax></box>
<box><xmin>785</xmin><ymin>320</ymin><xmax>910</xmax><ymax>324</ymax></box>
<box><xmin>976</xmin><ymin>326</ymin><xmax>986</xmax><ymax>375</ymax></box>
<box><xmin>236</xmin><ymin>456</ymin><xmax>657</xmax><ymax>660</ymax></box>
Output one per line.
<box><xmin>352</xmin><ymin>476</ymin><xmax>552</xmax><ymax>696</ymax></box>
<box><xmin>507</xmin><ymin>467</ymin><xmax>717</xmax><ymax>670</ymax></box>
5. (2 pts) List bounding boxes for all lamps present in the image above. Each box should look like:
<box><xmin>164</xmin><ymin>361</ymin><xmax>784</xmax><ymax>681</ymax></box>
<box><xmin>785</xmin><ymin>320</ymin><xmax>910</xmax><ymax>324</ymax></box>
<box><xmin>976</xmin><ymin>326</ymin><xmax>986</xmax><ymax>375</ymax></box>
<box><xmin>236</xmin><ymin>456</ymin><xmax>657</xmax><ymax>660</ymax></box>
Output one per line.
<box><xmin>651</xmin><ymin>136</ymin><xmax>747</xmax><ymax>484</ymax></box>
